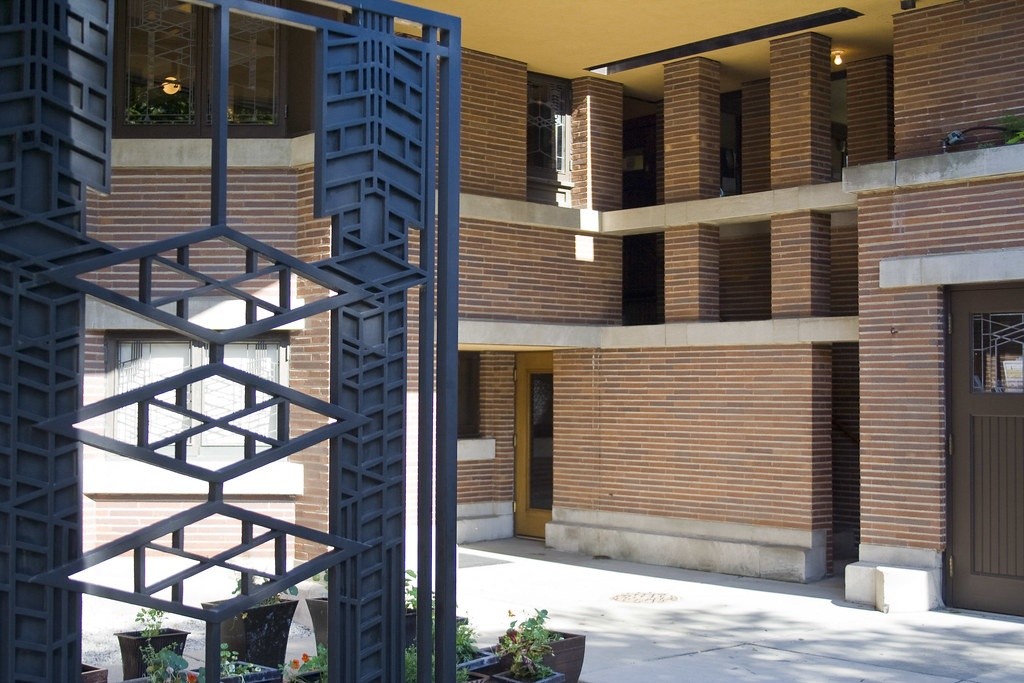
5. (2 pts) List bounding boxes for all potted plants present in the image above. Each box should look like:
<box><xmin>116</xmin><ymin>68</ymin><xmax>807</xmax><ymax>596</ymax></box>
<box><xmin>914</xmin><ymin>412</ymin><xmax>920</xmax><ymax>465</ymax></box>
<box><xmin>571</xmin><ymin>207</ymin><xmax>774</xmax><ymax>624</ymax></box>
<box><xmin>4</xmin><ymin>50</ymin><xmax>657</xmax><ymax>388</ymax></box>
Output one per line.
<box><xmin>497</xmin><ymin>608</ymin><xmax>586</xmax><ymax>683</ymax></box>
<box><xmin>404</xmin><ymin>569</ymin><xmax>417</xmax><ymax>648</ymax></box>
<box><xmin>304</xmin><ymin>568</ymin><xmax>328</xmax><ymax>656</ymax></box>
<box><xmin>192</xmin><ymin>642</ymin><xmax>282</xmax><ymax>683</ymax></box>
<box><xmin>201</xmin><ymin>569</ymin><xmax>299</xmax><ymax>670</ymax></box>
<box><xmin>113</xmin><ymin>608</ymin><xmax>192</xmax><ymax>680</ymax></box>
<box><xmin>493</xmin><ymin>651</ymin><xmax>567</xmax><ymax>683</ymax></box>
<box><xmin>119</xmin><ymin>641</ymin><xmax>201</xmax><ymax>683</ymax></box>
<box><xmin>403</xmin><ymin>651</ymin><xmax>491</xmax><ymax>683</ymax></box>
<box><xmin>430</xmin><ymin>621</ymin><xmax>501</xmax><ymax>670</ymax></box>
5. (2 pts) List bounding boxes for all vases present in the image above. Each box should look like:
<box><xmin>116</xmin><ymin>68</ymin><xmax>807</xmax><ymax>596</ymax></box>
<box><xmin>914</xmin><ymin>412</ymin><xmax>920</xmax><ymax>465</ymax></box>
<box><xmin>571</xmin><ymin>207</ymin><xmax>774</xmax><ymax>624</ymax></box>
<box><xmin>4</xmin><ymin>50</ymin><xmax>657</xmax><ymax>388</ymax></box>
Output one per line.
<box><xmin>291</xmin><ymin>669</ymin><xmax>323</xmax><ymax>683</ymax></box>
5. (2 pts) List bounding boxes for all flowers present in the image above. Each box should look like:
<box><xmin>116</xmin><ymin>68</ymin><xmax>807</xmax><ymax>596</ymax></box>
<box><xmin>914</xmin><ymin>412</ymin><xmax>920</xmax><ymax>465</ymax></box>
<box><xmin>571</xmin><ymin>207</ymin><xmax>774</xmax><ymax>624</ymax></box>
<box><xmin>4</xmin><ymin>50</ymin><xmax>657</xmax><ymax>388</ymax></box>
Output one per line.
<box><xmin>277</xmin><ymin>642</ymin><xmax>327</xmax><ymax>683</ymax></box>
